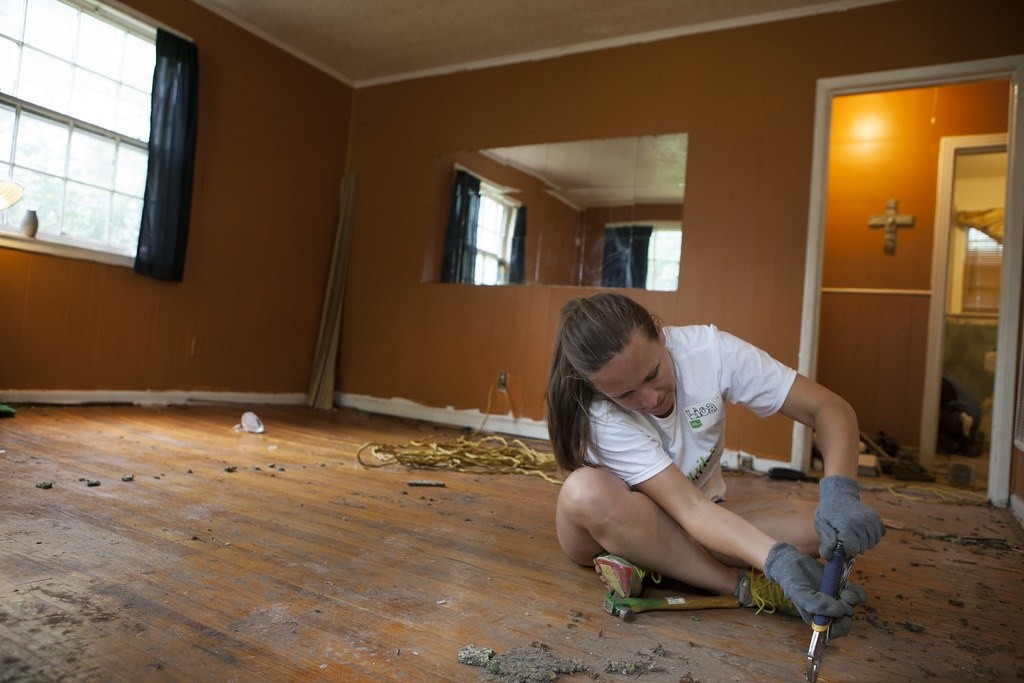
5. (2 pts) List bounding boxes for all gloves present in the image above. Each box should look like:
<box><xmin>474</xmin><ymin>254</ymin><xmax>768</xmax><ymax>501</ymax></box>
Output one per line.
<box><xmin>765</xmin><ymin>541</ymin><xmax>869</xmax><ymax>641</ymax></box>
<box><xmin>812</xmin><ymin>475</ymin><xmax>887</xmax><ymax>558</ymax></box>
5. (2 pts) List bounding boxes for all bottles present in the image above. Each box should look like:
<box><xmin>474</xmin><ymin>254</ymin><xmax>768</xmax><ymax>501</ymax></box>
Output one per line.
<box><xmin>22</xmin><ymin>210</ymin><xmax>38</xmax><ymax>237</ymax></box>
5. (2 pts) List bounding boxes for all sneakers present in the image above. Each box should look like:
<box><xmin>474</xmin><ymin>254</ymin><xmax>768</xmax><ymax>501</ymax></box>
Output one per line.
<box><xmin>736</xmin><ymin>565</ymin><xmax>805</xmax><ymax>618</ymax></box>
<box><xmin>593</xmin><ymin>548</ymin><xmax>666</xmax><ymax>600</ymax></box>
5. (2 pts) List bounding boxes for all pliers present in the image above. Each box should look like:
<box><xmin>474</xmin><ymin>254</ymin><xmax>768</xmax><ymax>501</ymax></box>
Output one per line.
<box><xmin>804</xmin><ymin>541</ymin><xmax>856</xmax><ymax>683</ymax></box>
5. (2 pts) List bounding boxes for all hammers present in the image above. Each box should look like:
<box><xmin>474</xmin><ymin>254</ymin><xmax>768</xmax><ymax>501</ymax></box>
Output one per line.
<box><xmin>603</xmin><ymin>589</ymin><xmax>740</xmax><ymax>623</ymax></box>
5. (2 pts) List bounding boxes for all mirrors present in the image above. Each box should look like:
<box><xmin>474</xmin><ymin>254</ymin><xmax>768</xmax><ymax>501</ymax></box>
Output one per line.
<box><xmin>419</xmin><ymin>132</ymin><xmax>690</xmax><ymax>292</ymax></box>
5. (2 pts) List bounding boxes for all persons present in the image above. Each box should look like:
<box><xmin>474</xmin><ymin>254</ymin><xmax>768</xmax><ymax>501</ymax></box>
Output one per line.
<box><xmin>546</xmin><ymin>293</ymin><xmax>884</xmax><ymax>638</ymax></box>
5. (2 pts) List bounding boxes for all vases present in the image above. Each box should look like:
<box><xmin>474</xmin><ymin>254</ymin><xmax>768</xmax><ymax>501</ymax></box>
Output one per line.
<box><xmin>19</xmin><ymin>208</ymin><xmax>38</xmax><ymax>237</ymax></box>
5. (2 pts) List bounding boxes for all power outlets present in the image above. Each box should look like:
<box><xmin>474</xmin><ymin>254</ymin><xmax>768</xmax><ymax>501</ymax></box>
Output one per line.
<box><xmin>189</xmin><ymin>337</ymin><xmax>201</xmax><ymax>357</ymax></box>
